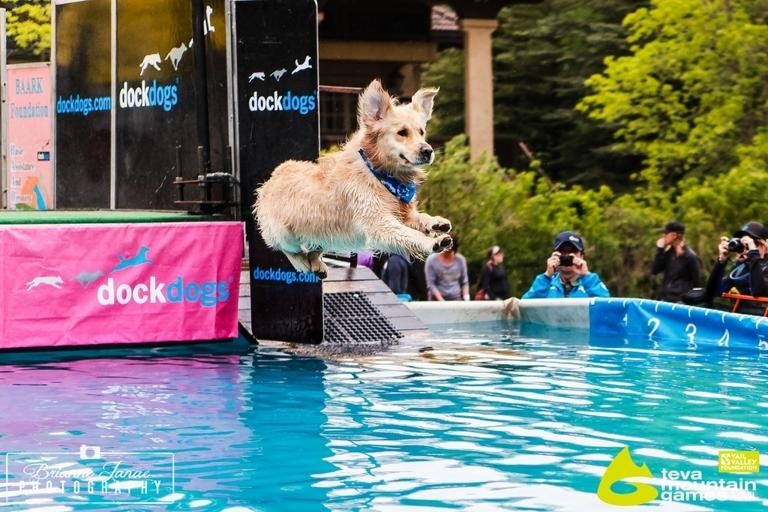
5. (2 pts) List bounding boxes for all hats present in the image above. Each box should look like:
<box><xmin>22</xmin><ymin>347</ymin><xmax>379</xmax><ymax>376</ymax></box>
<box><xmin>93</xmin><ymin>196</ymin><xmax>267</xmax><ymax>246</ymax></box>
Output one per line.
<box><xmin>733</xmin><ymin>221</ymin><xmax>766</xmax><ymax>241</ymax></box>
<box><xmin>658</xmin><ymin>222</ymin><xmax>685</xmax><ymax>234</ymax></box>
<box><xmin>552</xmin><ymin>230</ymin><xmax>584</xmax><ymax>252</ymax></box>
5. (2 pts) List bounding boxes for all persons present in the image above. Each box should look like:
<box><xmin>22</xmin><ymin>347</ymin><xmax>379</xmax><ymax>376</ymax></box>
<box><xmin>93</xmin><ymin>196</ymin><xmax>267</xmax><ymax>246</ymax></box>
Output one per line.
<box><xmin>475</xmin><ymin>245</ymin><xmax>512</xmax><ymax>301</ymax></box>
<box><xmin>339</xmin><ymin>230</ymin><xmax>471</xmax><ymax>302</ymax></box>
<box><xmin>520</xmin><ymin>230</ymin><xmax>611</xmax><ymax>297</ymax></box>
<box><xmin>704</xmin><ymin>221</ymin><xmax>768</xmax><ymax>312</ymax></box>
<box><xmin>647</xmin><ymin>219</ymin><xmax>702</xmax><ymax>304</ymax></box>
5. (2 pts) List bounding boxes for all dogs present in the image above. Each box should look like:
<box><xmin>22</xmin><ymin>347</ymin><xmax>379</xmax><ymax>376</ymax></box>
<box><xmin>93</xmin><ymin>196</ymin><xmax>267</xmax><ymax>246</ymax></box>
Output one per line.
<box><xmin>249</xmin><ymin>77</ymin><xmax>456</xmax><ymax>280</ymax></box>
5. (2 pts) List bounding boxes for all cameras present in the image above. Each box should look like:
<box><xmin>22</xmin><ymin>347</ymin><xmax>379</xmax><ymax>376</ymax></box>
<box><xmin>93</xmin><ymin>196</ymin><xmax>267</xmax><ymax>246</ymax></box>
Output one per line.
<box><xmin>727</xmin><ymin>238</ymin><xmax>744</xmax><ymax>253</ymax></box>
<box><xmin>559</xmin><ymin>255</ymin><xmax>573</xmax><ymax>266</ymax></box>
